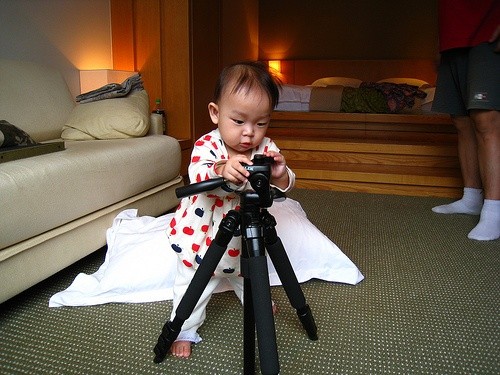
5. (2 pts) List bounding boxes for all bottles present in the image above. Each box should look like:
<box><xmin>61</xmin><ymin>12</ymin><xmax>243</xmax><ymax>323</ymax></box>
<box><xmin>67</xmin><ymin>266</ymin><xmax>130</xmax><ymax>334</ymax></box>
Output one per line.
<box><xmin>151</xmin><ymin>99</ymin><xmax>166</xmax><ymax>135</ymax></box>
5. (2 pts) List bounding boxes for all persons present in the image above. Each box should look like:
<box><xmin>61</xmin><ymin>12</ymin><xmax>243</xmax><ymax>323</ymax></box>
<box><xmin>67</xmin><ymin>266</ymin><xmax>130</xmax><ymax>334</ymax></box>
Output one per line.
<box><xmin>431</xmin><ymin>0</ymin><xmax>500</xmax><ymax>242</ymax></box>
<box><xmin>165</xmin><ymin>59</ymin><xmax>296</xmax><ymax>357</ymax></box>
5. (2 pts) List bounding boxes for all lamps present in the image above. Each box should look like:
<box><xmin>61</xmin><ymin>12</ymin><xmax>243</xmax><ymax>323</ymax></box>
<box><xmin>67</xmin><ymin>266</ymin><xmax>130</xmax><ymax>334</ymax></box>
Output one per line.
<box><xmin>78</xmin><ymin>69</ymin><xmax>137</xmax><ymax>95</ymax></box>
<box><xmin>267</xmin><ymin>61</ymin><xmax>282</xmax><ymax>78</ymax></box>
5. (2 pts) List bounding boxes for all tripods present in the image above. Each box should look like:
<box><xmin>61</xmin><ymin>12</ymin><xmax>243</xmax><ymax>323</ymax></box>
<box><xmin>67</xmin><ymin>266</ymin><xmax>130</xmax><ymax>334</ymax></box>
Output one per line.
<box><xmin>153</xmin><ymin>154</ymin><xmax>318</xmax><ymax>375</ymax></box>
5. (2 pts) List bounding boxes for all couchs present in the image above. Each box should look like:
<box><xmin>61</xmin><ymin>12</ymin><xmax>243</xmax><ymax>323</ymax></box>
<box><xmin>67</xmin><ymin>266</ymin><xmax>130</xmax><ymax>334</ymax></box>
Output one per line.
<box><xmin>0</xmin><ymin>60</ymin><xmax>183</xmax><ymax>303</ymax></box>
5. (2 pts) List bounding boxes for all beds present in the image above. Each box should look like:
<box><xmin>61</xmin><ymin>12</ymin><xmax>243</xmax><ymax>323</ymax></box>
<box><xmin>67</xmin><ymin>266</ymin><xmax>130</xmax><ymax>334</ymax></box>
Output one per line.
<box><xmin>256</xmin><ymin>57</ymin><xmax>464</xmax><ymax>198</ymax></box>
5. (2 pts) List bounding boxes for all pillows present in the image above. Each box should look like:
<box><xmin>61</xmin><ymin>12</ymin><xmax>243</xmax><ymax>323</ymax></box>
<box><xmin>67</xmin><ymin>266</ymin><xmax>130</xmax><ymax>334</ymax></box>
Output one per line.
<box><xmin>61</xmin><ymin>87</ymin><xmax>150</xmax><ymax>140</ymax></box>
<box><xmin>377</xmin><ymin>78</ymin><xmax>429</xmax><ymax>88</ymax></box>
<box><xmin>311</xmin><ymin>77</ymin><xmax>363</xmax><ymax>87</ymax></box>
